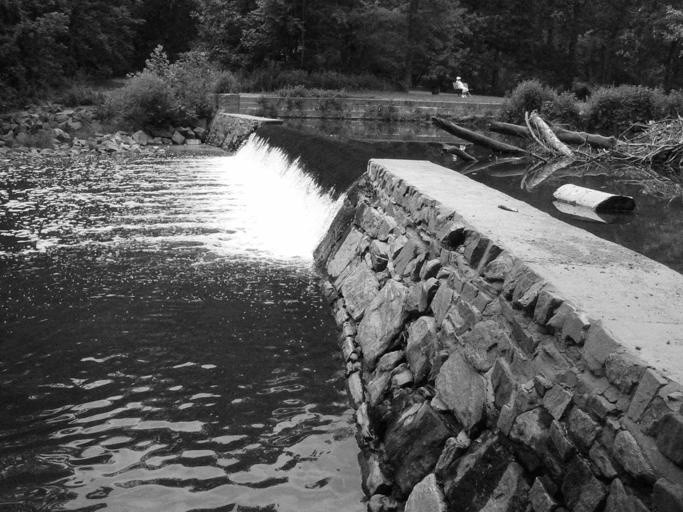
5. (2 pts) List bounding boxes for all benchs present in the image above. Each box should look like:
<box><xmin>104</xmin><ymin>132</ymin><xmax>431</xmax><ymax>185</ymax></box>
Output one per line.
<box><xmin>452</xmin><ymin>82</ymin><xmax>474</xmax><ymax>96</ymax></box>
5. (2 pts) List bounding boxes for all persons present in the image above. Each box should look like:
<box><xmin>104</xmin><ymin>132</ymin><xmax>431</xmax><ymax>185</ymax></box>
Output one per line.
<box><xmin>455</xmin><ymin>75</ymin><xmax>469</xmax><ymax>99</ymax></box>
